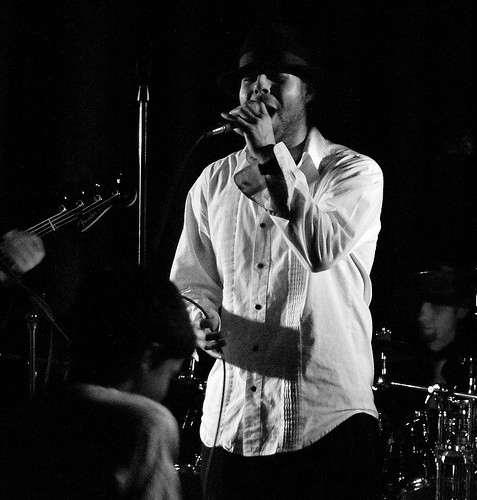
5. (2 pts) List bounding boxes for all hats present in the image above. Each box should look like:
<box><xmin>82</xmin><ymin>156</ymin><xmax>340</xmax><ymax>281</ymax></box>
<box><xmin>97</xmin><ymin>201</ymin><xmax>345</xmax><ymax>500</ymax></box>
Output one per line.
<box><xmin>237</xmin><ymin>47</ymin><xmax>310</xmax><ymax>77</ymax></box>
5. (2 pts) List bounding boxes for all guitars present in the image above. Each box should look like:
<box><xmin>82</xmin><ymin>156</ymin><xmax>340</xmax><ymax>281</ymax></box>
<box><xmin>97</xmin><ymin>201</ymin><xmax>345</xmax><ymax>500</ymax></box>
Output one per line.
<box><xmin>0</xmin><ymin>170</ymin><xmax>137</xmax><ymax>281</ymax></box>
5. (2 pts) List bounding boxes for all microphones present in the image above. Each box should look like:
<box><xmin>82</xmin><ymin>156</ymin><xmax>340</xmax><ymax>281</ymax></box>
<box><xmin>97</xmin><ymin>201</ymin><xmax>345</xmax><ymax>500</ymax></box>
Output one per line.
<box><xmin>203</xmin><ymin>121</ymin><xmax>241</xmax><ymax>138</ymax></box>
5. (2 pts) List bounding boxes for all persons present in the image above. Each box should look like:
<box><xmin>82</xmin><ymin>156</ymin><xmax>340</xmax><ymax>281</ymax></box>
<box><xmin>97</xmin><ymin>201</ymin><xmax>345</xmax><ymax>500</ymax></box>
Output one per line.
<box><xmin>163</xmin><ymin>26</ymin><xmax>386</xmax><ymax>500</ymax></box>
<box><xmin>374</xmin><ymin>268</ymin><xmax>477</xmax><ymax>500</ymax></box>
<box><xmin>0</xmin><ymin>229</ymin><xmax>194</xmax><ymax>500</ymax></box>
